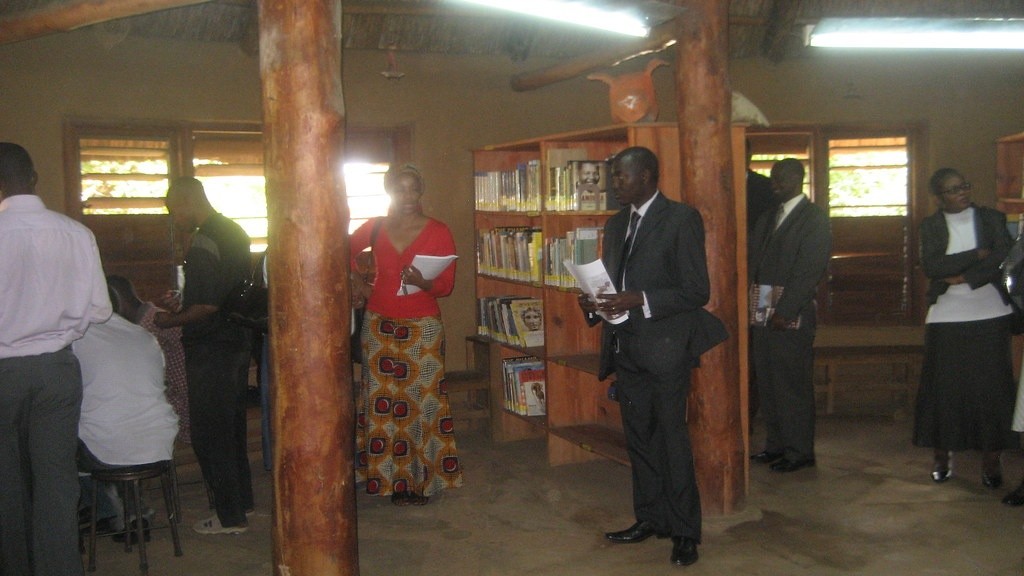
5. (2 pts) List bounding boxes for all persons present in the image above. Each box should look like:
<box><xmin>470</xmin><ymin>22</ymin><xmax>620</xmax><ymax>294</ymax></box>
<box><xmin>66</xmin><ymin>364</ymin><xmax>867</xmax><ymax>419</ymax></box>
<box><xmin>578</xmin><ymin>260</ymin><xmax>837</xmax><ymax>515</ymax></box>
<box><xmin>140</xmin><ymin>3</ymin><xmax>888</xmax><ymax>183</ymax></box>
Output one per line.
<box><xmin>578</xmin><ymin>146</ymin><xmax>731</xmax><ymax>566</ymax></box>
<box><xmin>1002</xmin><ymin>234</ymin><xmax>1024</xmax><ymax>505</ymax></box>
<box><xmin>918</xmin><ymin>168</ymin><xmax>1024</xmax><ymax>488</ymax></box>
<box><xmin>77</xmin><ymin>276</ymin><xmax>193</xmax><ymax>535</ymax></box>
<box><xmin>0</xmin><ymin>141</ymin><xmax>112</xmax><ymax>576</ymax></box>
<box><xmin>71</xmin><ymin>312</ymin><xmax>181</xmax><ymax>543</ymax></box>
<box><xmin>154</xmin><ymin>178</ymin><xmax>257</xmax><ymax>535</ymax></box>
<box><xmin>350</xmin><ymin>163</ymin><xmax>464</xmax><ymax>506</ymax></box>
<box><xmin>576</xmin><ymin>162</ymin><xmax>600</xmax><ymax>194</ymax></box>
<box><xmin>744</xmin><ymin>140</ymin><xmax>832</xmax><ymax>472</ymax></box>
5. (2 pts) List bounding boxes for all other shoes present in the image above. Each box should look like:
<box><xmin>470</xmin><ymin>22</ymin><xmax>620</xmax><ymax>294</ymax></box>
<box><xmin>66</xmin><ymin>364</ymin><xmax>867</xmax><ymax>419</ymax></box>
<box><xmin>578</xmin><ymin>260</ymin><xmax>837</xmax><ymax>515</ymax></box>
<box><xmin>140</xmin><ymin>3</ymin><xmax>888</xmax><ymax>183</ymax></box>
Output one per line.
<box><xmin>113</xmin><ymin>517</ymin><xmax>150</xmax><ymax>544</ymax></box>
<box><xmin>81</xmin><ymin>517</ymin><xmax>125</xmax><ymax>535</ymax></box>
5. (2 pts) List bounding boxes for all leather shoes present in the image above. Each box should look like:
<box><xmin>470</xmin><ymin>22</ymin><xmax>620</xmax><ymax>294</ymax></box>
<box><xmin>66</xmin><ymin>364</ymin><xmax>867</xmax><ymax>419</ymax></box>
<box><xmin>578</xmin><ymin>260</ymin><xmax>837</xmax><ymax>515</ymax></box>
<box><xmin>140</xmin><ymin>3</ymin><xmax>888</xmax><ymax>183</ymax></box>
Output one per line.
<box><xmin>1003</xmin><ymin>476</ymin><xmax>1024</xmax><ymax>505</ymax></box>
<box><xmin>607</xmin><ymin>521</ymin><xmax>670</xmax><ymax>544</ymax></box>
<box><xmin>671</xmin><ymin>536</ymin><xmax>697</xmax><ymax>566</ymax></box>
<box><xmin>750</xmin><ymin>448</ymin><xmax>781</xmax><ymax>462</ymax></box>
<box><xmin>768</xmin><ymin>458</ymin><xmax>815</xmax><ymax>472</ymax></box>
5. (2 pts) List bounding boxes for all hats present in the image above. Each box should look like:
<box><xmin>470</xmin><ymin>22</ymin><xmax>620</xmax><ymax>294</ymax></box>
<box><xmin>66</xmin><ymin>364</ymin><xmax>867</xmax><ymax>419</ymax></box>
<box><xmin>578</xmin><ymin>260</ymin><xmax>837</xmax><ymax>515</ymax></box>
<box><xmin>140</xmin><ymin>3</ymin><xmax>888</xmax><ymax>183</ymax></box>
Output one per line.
<box><xmin>383</xmin><ymin>161</ymin><xmax>422</xmax><ymax>194</ymax></box>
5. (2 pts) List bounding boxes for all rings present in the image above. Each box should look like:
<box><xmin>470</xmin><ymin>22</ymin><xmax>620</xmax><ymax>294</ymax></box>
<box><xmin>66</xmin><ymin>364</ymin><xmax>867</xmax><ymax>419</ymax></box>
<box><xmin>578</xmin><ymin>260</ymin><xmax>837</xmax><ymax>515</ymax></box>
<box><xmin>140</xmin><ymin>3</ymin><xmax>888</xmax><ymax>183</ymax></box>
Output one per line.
<box><xmin>612</xmin><ymin>305</ymin><xmax>615</xmax><ymax>311</ymax></box>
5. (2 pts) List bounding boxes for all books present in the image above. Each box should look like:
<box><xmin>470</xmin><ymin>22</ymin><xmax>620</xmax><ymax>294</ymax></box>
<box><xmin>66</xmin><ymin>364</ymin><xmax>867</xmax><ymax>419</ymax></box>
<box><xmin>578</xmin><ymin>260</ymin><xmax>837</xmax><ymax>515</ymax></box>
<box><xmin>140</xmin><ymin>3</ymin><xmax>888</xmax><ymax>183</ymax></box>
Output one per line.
<box><xmin>502</xmin><ymin>356</ymin><xmax>546</xmax><ymax>416</ymax></box>
<box><xmin>476</xmin><ymin>225</ymin><xmax>542</xmax><ymax>281</ymax></box>
<box><xmin>750</xmin><ymin>284</ymin><xmax>801</xmax><ymax>330</ymax></box>
<box><xmin>474</xmin><ymin>160</ymin><xmax>541</xmax><ymax>211</ymax></box>
<box><xmin>564</xmin><ymin>259</ymin><xmax>628</xmax><ymax>325</ymax></box>
<box><xmin>478</xmin><ymin>295</ymin><xmax>544</xmax><ymax>347</ymax></box>
<box><xmin>397</xmin><ymin>255</ymin><xmax>459</xmax><ymax>297</ymax></box>
<box><xmin>545</xmin><ymin>228</ymin><xmax>604</xmax><ymax>288</ymax></box>
<box><xmin>546</xmin><ymin>148</ymin><xmax>623</xmax><ymax>211</ymax></box>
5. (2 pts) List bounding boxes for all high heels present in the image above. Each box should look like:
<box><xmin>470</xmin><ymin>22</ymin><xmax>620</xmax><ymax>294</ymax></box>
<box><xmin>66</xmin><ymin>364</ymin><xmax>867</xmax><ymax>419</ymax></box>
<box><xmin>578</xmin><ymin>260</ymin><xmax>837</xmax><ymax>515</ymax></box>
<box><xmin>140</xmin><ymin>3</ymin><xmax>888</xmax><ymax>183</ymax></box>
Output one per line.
<box><xmin>982</xmin><ymin>458</ymin><xmax>1003</xmax><ymax>488</ymax></box>
<box><xmin>932</xmin><ymin>451</ymin><xmax>951</xmax><ymax>482</ymax></box>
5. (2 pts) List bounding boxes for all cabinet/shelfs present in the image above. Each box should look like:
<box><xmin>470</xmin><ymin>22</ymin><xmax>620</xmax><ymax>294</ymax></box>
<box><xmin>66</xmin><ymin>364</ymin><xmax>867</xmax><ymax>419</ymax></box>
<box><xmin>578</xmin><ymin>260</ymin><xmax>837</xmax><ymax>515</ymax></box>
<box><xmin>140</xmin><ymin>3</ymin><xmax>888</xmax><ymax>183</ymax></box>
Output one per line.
<box><xmin>472</xmin><ymin>121</ymin><xmax>683</xmax><ymax>471</ymax></box>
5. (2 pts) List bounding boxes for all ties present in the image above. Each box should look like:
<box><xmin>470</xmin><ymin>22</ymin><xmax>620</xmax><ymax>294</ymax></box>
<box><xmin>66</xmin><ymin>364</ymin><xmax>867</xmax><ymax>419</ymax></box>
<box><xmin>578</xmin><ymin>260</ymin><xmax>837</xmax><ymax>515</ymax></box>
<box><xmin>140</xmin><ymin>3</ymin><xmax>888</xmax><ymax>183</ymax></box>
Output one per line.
<box><xmin>616</xmin><ymin>211</ymin><xmax>641</xmax><ymax>292</ymax></box>
<box><xmin>767</xmin><ymin>207</ymin><xmax>784</xmax><ymax>241</ymax></box>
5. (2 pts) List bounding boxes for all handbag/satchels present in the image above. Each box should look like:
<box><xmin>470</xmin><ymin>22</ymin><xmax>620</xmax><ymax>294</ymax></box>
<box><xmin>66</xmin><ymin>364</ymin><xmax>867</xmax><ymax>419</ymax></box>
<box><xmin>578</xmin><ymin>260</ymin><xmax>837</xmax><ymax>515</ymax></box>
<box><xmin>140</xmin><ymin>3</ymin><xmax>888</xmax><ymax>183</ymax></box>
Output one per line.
<box><xmin>350</xmin><ymin>305</ymin><xmax>364</xmax><ymax>364</ymax></box>
<box><xmin>221</xmin><ymin>252</ymin><xmax>269</xmax><ymax>335</ymax></box>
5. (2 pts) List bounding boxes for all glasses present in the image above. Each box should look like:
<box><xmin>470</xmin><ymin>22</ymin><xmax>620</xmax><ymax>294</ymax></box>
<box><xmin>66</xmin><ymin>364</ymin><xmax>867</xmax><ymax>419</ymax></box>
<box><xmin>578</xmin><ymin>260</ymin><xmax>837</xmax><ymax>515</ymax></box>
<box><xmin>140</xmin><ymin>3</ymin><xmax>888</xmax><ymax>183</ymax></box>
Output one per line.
<box><xmin>937</xmin><ymin>182</ymin><xmax>972</xmax><ymax>196</ymax></box>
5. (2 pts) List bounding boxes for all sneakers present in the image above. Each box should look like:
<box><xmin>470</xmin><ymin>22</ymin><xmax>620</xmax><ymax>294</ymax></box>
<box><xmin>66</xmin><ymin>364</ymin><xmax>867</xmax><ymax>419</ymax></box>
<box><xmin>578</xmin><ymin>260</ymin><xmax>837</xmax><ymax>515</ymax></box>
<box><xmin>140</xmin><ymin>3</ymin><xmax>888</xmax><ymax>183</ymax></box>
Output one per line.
<box><xmin>193</xmin><ymin>513</ymin><xmax>249</xmax><ymax>534</ymax></box>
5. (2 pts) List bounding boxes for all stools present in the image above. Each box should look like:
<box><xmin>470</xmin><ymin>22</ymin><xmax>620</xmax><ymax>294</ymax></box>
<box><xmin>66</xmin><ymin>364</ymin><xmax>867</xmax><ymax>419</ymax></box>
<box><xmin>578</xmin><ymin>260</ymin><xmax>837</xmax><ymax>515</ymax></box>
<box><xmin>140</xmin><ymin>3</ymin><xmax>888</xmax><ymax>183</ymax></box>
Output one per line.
<box><xmin>87</xmin><ymin>462</ymin><xmax>183</xmax><ymax>576</ymax></box>
